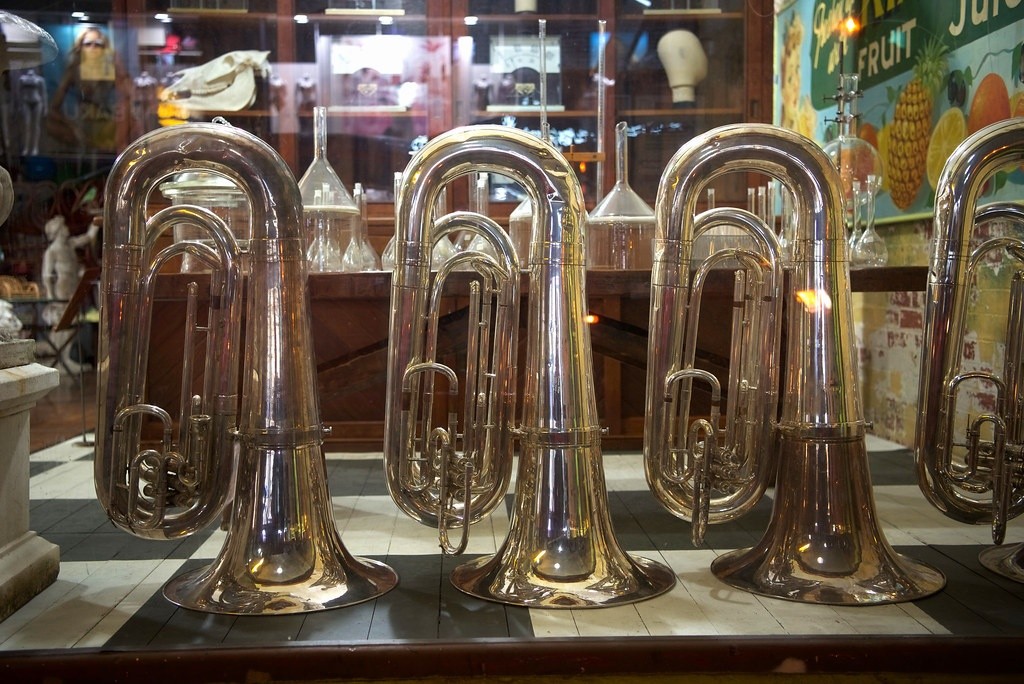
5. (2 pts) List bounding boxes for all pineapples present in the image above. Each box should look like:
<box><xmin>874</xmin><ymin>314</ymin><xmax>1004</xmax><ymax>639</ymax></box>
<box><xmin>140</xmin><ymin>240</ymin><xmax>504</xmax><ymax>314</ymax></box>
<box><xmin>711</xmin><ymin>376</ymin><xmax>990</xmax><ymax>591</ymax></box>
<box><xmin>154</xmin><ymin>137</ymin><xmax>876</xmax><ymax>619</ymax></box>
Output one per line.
<box><xmin>884</xmin><ymin>32</ymin><xmax>950</xmax><ymax>211</ymax></box>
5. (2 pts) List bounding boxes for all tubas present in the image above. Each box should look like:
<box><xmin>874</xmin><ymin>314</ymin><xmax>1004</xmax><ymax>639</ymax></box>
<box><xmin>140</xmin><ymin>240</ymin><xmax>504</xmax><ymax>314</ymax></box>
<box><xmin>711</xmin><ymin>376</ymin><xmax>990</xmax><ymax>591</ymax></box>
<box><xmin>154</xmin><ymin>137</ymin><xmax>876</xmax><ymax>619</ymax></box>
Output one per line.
<box><xmin>641</xmin><ymin>120</ymin><xmax>948</xmax><ymax>606</ymax></box>
<box><xmin>88</xmin><ymin>122</ymin><xmax>400</xmax><ymax>619</ymax></box>
<box><xmin>912</xmin><ymin>112</ymin><xmax>1024</xmax><ymax>589</ymax></box>
<box><xmin>379</xmin><ymin>121</ymin><xmax>680</xmax><ymax>615</ymax></box>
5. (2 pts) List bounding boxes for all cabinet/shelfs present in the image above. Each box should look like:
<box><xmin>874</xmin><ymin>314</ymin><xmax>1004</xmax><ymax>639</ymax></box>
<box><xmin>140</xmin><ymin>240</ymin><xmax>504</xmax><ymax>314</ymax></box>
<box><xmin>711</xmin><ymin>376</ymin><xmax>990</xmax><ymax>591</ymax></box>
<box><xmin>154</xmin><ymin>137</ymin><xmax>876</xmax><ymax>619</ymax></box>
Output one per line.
<box><xmin>0</xmin><ymin>0</ymin><xmax>774</xmax><ymax>269</ymax></box>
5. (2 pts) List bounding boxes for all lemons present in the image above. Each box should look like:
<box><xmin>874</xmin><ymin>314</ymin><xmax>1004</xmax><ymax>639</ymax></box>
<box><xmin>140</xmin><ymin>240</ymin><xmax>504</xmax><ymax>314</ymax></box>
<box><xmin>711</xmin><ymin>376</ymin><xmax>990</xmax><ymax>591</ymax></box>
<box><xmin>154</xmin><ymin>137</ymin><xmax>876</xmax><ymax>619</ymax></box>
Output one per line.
<box><xmin>831</xmin><ymin>72</ymin><xmax>1024</xmax><ymax>197</ymax></box>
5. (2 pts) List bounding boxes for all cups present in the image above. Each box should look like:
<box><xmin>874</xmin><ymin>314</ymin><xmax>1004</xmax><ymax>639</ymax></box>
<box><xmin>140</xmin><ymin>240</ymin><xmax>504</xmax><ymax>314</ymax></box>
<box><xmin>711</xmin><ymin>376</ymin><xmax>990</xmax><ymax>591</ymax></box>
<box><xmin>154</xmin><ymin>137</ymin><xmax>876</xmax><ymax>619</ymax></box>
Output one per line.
<box><xmin>160</xmin><ymin>172</ymin><xmax>254</xmax><ymax>247</ymax></box>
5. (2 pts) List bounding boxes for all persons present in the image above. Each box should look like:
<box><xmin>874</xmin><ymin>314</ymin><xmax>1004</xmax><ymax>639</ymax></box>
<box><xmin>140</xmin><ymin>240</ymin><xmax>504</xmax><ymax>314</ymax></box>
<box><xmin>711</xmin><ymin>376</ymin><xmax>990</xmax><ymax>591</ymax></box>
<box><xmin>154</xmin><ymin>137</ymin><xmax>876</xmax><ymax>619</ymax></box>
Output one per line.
<box><xmin>132</xmin><ymin>72</ymin><xmax>316</xmax><ymax>132</ymax></box>
<box><xmin>47</xmin><ymin>27</ymin><xmax>148</xmax><ymax>153</ymax></box>
<box><xmin>472</xmin><ymin>72</ymin><xmax>520</xmax><ymax>121</ymax></box>
<box><xmin>17</xmin><ymin>70</ymin><xmax>48</xmax><ymax>156</ymax></box>
<box><xmin>41</xmin><ymin>215</ymin><xmax>102</xmax><ymax>373</ymax></box>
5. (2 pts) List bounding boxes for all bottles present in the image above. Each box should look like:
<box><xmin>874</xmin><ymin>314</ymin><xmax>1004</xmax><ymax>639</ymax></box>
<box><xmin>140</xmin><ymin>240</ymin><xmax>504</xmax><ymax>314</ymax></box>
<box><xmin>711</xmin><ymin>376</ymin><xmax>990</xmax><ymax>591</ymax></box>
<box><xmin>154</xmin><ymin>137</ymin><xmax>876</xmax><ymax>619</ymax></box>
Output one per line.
<box><xmin>309</xmin><ymin>169</ymin><xmax>500</xmax><ymax>275</ymax></box>
<box><xmin>705</xmin><ymin>177</ymin><xmax>801</xmax><ymax>264</ymax></box>
<box><xmin>848</xmin><ymin>173</ymin><xmax>888</xmax><ymax>269</ymax></box>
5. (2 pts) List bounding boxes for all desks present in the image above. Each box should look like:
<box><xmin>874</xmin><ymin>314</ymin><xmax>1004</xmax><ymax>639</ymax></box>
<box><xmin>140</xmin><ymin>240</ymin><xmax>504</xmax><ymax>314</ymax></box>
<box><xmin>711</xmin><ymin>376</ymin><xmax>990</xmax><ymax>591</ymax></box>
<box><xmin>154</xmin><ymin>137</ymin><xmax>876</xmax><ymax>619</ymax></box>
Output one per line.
<box><xmin>7</xmin><ymin>298</ymin><xmax>73</xmax><ymax>377</ymax></box>
